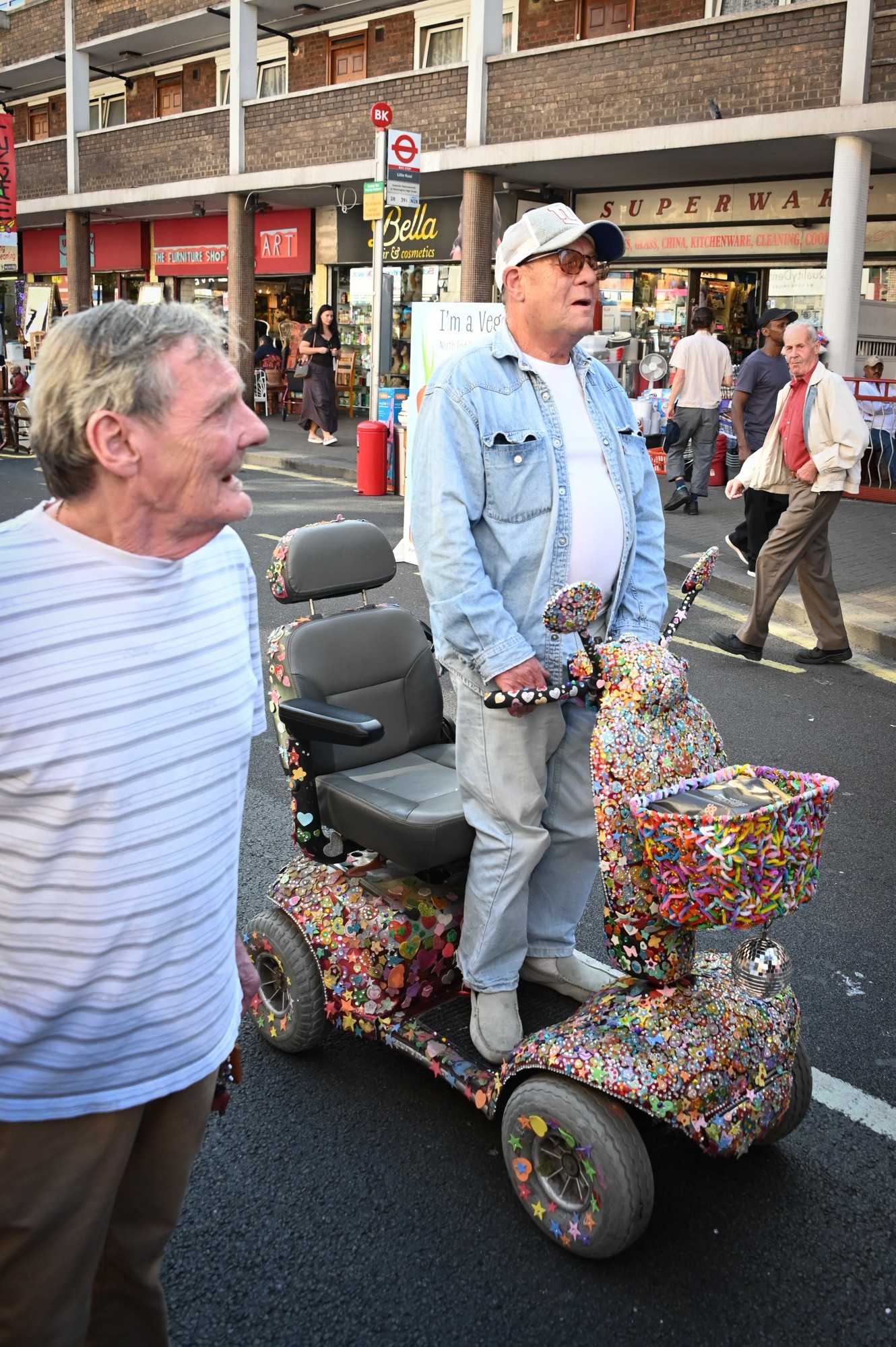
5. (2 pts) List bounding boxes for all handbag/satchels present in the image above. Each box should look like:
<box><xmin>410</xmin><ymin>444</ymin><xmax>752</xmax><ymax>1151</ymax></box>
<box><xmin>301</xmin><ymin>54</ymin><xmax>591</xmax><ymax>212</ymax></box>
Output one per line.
<box><xmin>294</xmin><ymin>363</ymin><xmax>311</xmax><ymax>377</ymax></box>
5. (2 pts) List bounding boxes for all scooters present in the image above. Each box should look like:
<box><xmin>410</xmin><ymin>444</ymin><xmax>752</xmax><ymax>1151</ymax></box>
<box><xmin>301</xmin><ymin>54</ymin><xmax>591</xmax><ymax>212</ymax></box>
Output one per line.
<box><xmin>232</xmin><ymin>511</ymin><xmax>843</xmax><ymax>1268</ymax></box>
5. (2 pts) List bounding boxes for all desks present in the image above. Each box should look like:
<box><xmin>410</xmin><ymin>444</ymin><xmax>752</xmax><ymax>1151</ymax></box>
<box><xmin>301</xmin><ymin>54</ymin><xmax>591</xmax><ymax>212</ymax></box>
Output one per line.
<box><xmin>256</xmin><ymin>384</ymin><xmax>292</xmax><ymax>415</ymax></box>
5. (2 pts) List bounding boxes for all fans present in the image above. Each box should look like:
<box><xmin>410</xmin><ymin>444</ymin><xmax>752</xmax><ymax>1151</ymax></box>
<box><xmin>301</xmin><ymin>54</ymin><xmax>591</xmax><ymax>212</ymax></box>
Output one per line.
<box><xmin>639</xmin><ymin>353</ymin><xmax>668</xmax><ymax>391</ymax></box>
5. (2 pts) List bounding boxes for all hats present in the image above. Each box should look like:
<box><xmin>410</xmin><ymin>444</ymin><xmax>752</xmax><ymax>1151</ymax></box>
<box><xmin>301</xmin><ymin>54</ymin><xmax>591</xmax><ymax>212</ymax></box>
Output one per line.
<box><xmin>662</xmin><ymin>419</ymin><xmax>680</xmax><ymax>454</ymax></box>
<box><xmin>757</xmin><ymin>308</ymin><xmax>798</xmax><ymax>333</ymax></box>
<box><xmin>495</xmin><ymin>203</ymin><xmax>626</xmax><ymax>294</ymax></box>
<box><xmin>864</xmin><ymin>355</ymin><xmax>884</xmax><ymax>369</ymax></box>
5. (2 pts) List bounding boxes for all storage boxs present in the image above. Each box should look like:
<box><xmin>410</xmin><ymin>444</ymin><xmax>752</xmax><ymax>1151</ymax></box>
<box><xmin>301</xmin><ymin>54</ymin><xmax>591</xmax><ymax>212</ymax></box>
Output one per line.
<box><xmin>602</xmin><ymin>361</ymin><xmax>621</xmax><ymax>380</ymax></box>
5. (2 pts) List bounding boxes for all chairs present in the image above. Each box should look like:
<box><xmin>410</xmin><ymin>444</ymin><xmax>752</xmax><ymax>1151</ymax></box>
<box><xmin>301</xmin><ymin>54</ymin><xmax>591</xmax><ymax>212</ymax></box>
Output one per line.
<box><xmin>277</xmin><ymin>512</ymin><xmax>476</xmax><ymax>871</ymax></box>
<box><xmin>331</xmin><ymin>353</ymin><xmax>356</xmax><ymax>418</ymax></box>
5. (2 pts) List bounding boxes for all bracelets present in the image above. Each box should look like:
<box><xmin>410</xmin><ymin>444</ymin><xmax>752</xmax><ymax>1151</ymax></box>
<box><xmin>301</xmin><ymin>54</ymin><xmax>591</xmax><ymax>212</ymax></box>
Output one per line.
<box><xmin>237</xmin><ymin>958</ymin><xmax>252</xmax><ymax>968</ymax></box>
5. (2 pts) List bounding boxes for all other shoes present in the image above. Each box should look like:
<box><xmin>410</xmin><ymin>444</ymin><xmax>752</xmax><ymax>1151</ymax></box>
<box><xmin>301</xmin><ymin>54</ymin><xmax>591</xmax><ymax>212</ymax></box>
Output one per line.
<box><xmin>519</xmin><ymin>947</ymin><xmax>618</xmax><ymax>1004</ymax></box>
<box><xmin>308</xmin><ymin>436</ymin><xmax>323</xmax><ymax>443</ymax></box>
<box><xmin>323</xmin><ymin>436</ymin><xmax>338</xmax><ymax>445</ymax></box>
<box><xmin>469</xmin><ymin>983</ymin><xmax>524</xmax><ymax>1061</ymax></box>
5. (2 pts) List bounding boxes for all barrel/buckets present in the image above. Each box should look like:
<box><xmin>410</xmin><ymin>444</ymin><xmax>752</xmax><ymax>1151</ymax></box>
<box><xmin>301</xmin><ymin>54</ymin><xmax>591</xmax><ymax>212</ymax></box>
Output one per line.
<box><xmin>708</xmin><ymin>434</ymin><xmax>740</xmax><ymax>486</ymax></box>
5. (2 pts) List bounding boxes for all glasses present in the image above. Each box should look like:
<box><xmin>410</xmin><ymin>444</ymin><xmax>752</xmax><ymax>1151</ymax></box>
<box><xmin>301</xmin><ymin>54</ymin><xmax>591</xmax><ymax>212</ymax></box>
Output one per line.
<box><xmin>865</xmin><ymin>365</ymin><xmax>883</xmax><ymax>371</ymax></box>
<box><xmin>517</xmin><ymin>246</ymin><xmax>610</xmax><ymax>281</ymax></box>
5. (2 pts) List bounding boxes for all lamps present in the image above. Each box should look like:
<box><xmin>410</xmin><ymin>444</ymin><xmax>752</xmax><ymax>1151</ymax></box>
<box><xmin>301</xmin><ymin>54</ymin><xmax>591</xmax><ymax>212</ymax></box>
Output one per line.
<box><xmin>254</xmin><ymin>197</ymin><xmax>272</xmax><ymax>214</ymax></box>
<box><xmin>119</xmin><ymin>51</ymin><xmax>141</xmax><ymax>60</ymax></box>
<box><xmin>294</xmin><ymin>4</ymin><xmax>319</xmax><ymax>14</ymax></box>
<box><xmin>794</xmin><ymin>219</ymin><xmax>812</xmax><ymax>228</ymax></box>
<box><xmin>193</xmin><ymin>200</ymin><xmax>206</xmax><ymax>218</ymax></box>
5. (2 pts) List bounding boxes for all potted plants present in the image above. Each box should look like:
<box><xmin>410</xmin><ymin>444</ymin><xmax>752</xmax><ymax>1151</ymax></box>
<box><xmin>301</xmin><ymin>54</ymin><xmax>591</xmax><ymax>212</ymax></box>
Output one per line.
<box><xmin>262</xmin><ymin>355</ymin><xmax>283</xmax><ymax>382</ymax></box>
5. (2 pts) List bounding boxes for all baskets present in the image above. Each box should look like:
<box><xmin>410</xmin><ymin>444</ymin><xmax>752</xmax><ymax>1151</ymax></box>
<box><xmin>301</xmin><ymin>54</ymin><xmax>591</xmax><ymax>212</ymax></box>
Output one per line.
<box><xmin>629</xmin><ymin>763</ymin><xmax>839</xmax><ymax>932</ymax></box>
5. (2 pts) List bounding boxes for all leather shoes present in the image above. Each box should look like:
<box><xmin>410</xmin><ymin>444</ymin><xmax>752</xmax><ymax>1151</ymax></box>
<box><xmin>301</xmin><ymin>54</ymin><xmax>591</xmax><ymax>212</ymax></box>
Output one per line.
<box><xmin>684</xmin><ymin>501</ymin><xmax>699</xmax><ymax>515</ymax></box>
<box><xmin>709</xmin><ymin>632</ymin><xmax>763</xmax><ymax>661</ymax></box>
<box><xmin>664</xmin><ymin>486</ymin><xmax>691</xmax><ymax>510</ymax></box>
<box><xmin>794</xmin><ymin>647</ymin><xmax>852</xmax><ymax>664</ymax></box>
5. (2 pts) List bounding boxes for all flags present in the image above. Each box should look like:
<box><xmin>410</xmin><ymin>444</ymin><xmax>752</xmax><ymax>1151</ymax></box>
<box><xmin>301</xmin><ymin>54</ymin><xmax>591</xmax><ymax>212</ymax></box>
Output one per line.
<box><xmin>0</xmin><ymin>112</ymin><xmax>17</xmax><ymax>233</ymax></box>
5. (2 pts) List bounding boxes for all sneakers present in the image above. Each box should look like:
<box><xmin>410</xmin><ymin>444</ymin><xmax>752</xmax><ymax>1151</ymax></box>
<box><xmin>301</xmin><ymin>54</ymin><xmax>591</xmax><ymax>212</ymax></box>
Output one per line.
<box><xmin>746</xmin><ymin>563</ymin><xmax>756</xmax><ymax>577</ymax></box>
<box><xmin>725</xmin><ymin>532</ymin><xmax>750</xmax><ymax>565</ymax></box>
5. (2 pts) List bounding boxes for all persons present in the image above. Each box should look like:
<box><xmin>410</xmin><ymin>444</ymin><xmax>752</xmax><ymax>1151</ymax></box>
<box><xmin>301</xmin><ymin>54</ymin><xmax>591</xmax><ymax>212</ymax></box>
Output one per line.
<box><xmin>254</xmin><ymin>335</ymin><xmax>283</xmax><ymax>415</ymax></box>
<box><xmin>297</xmin><ymin>304</ymin><xmax>341</xmax><ymax>446</ymax></box>
<box><xmin>0</xmin><ymin>299</ymin><xmax>268</xmax><ymax>1347</ymax></box>
<box><xmin>663</xmin><ymin>307</ymin><xmax>733</xmax><ymax>515</ymax></box>
<box><xmin>849</xmin><ymin>355</ymin><xmax>896</xmax><ymax>482</ymax></box>
<box><xmin>404</xmin><ymin>205</ymin><xmax>671</xmax><ymax>1062</ymax></box>
<box><xmin>8</xmin><ymin>364</ymin><xmax>30</xmax><ymax>397</ymax></box>
<box><xmin>725</xmin><ymin>308</ymin><xmax>799</xmax><ymax>578</ymax></box>
<box><xmin>709</xmin><ymin>318</ymin><xmax>869</xmax><ymax>665</ymax></box>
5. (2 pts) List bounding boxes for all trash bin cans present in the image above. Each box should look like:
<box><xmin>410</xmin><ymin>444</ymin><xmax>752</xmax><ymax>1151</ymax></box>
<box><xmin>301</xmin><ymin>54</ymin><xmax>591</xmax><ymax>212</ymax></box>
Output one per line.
<box><xmin>393</xmin><ymin>422</ymin><xmax>407</xmax><ymax>496</ymax></box>
<box><xmin>353</xmin><ymin>420</ymin><xmax>388</xmax><ymax>496</ymax></box>
